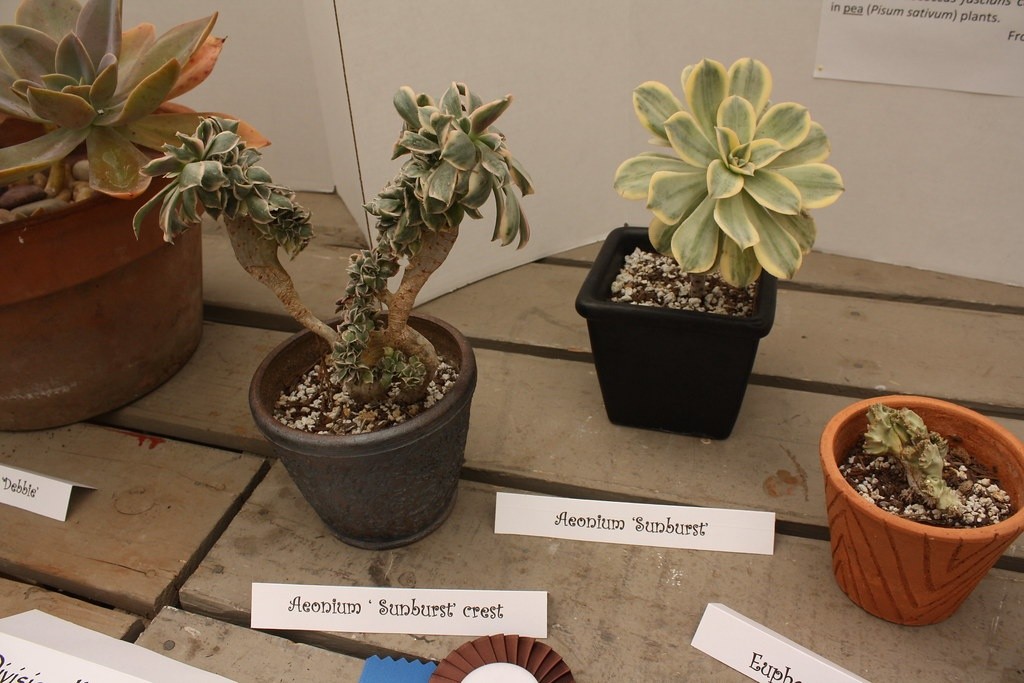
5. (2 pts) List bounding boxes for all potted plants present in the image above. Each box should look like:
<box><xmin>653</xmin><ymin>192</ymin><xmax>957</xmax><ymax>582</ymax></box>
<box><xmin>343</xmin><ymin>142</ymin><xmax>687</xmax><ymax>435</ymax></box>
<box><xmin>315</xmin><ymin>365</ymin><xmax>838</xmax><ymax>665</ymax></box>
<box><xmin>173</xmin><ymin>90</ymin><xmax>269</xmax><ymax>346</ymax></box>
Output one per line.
<box><xmin>133</xmin><ymin>81</ymin><xmax>540</xmax><ymax>553</ymax></box>
<box><xmin>816</xmin><ymin>394</ymin><xmax>1024</xmax><ymax>627</ymax></box>
<box><xmin>575</xmin><ymin>59</ymin><xmax>846</xmax><ymax>445</ymax></box>
<box><xmin>1</xmin><ymin>1</ymin><xmax>272</xmax><ymax>436</ymax></box>
